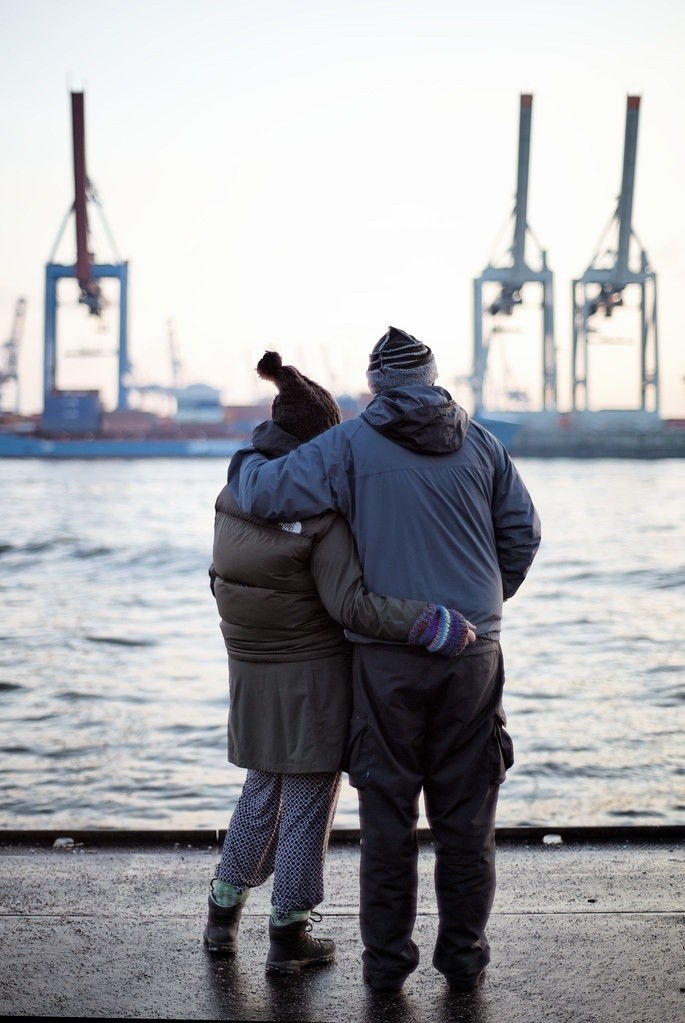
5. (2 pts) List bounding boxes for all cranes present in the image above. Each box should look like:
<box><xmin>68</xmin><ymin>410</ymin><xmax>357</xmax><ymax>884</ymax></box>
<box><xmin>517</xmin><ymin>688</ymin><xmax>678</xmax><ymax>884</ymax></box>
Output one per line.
<box><xmin>0</xmin><ymin>291</ymin><xmax>29</xmax><ymax>401</ymax></box>
<box><xmin>572</xmin><ymin>95</ymin><xmax>660</xmax><ymax>413</ymax></box>
<box><xmin>474</xmin><ymin>94</ymin><xmax>557</xmax><ymax>411</ymax></box>
<box><xmin>44</xmin><ymin>91</ymin><xmax>125</xmax><ymax>411</ymax></box>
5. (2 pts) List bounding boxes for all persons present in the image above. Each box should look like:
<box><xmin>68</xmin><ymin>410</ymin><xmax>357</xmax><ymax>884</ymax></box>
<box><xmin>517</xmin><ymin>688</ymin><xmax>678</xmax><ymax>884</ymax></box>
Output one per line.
<box><xmin>201</xmin><ymin>348</ymin><xmax>474</xmax><ymax>971</ymax></box>
<box><xmin>226</xmin><ymin>327</ymin><xmax>540</xmax><ymax>992</ymax></box>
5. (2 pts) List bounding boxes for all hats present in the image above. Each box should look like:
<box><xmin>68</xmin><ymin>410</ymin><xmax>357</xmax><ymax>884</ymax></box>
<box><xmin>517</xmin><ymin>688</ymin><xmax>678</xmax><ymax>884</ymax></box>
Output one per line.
<box><xmin>255</xmin><ymin>349</ymin><xmax>342</xmax><ymax>443</ymax></box>
<box><xmin>366</xmin><ymin>325</ymin><xmax>439</xmax><ymax>394</ymax></box>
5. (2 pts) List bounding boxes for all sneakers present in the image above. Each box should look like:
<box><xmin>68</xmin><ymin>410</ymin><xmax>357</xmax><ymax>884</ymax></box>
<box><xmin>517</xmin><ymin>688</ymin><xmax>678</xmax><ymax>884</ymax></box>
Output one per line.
<box><xmin>263</xmin><ymin>909</ymin><xmax>338</xmax><ymax>975</ymax></box>
<box><xmin>202</xmin><ymin>877</ymin><xmax>243</xmax><ymax>956</ymax></box>
